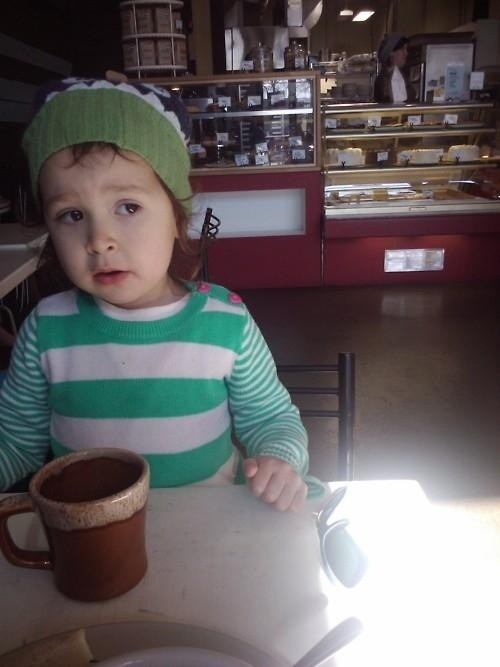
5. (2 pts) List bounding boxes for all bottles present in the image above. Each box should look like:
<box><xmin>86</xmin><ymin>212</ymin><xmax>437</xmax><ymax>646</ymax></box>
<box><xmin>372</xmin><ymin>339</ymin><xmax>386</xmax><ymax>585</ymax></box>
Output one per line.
<box><xmin>338</xmin><ymin>50</ymin><xmax>350</xmax><ymax>76</ymax></box>
<box><xmin>252</xmin><ymin>41</ymin><xmax>307</xmax><ymax>73</ymax></box>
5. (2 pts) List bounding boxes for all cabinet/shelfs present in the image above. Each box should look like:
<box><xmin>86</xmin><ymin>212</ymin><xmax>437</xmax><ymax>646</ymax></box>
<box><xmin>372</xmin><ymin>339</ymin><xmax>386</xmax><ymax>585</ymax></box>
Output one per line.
<box><xmin>117</xmin><ymin>0</ymin><xmax>190</xmax><ymax>78</ymax></box>
<box><xmin>321</xmin><ymin>98</ymin><xmax>500</xmax><ymax>218</ymax></box>
<box><xmin>130</xmin><ymin>70</ymin><xmax>321</xmax><ymax>175</ymax></box>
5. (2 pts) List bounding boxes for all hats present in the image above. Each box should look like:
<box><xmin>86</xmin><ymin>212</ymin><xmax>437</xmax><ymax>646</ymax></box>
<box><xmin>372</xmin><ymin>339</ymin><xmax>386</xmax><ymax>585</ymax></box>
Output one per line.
<box><xmin>22</xmin><ymin>75</ymin><xmax>193</xmax><ymax>216</ymax></box>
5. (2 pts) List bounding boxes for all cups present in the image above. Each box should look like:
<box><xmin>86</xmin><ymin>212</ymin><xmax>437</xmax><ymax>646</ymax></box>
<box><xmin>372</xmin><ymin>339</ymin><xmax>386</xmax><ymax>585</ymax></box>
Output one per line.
<box><xmin>0</xmin><ymin>447</ymin><xmax>149</xmax><ymax>601</ymax></box>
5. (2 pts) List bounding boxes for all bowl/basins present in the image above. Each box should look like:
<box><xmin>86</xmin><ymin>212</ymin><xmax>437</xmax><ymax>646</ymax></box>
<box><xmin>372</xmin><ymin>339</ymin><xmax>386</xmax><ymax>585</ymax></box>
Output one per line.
<box><xmin>343</xmin><ymin>82</ymin><xmax>371</xmax><ymax>98</ymax></box>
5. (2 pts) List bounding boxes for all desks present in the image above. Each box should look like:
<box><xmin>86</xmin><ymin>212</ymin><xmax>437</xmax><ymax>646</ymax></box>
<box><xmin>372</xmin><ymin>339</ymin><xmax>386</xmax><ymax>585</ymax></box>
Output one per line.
<box><xmin>0</xmin><ymin>477</ymin><xmax>497</xmax><ymax>666</ymax></box>
<box><xmin>0</xmin><ymin>224</ymin><xmax>54</xmax><ymax>348</ymax></box>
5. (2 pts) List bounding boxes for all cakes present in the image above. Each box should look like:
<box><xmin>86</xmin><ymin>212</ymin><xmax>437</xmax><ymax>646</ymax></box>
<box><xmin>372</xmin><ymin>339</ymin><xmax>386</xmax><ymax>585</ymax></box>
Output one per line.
<box><xmin>409</xmin><ymin>149</ymin><xmax>443</xmax><ymax>164</ymax></box>
<box><xmin>325</xmin><ymin>147</ymin><xmax>366</xmax><ymax>164</ymax></box>
<box><xmin>447</xmin><ymin>144</ymin><xmax>481</xmax><ymax>162</ymax></box>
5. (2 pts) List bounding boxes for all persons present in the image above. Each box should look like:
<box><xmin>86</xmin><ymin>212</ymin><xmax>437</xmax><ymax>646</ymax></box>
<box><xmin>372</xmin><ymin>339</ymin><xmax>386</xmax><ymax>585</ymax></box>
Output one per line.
<box><xmin>374</xmin><ymin>31</ymin><xmax>417</xmax><ymax>103</ymax></box>
<box><xmin>0</xmin><ymin>78</ymin><xmax>310</xmax><ymax>513</ymax></box>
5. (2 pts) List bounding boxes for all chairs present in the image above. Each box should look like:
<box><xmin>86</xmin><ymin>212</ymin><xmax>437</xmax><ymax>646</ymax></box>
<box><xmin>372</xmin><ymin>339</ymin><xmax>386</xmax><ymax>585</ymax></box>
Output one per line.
<box><xmin>276</xmin><ymin>352</ymin><xmax>355</xmax><ymax>481</ymax></box>
<box><xmin>187</xmin><ymin>209</ymin><xmax>221</xmax><ymax>282</ymax></box>
